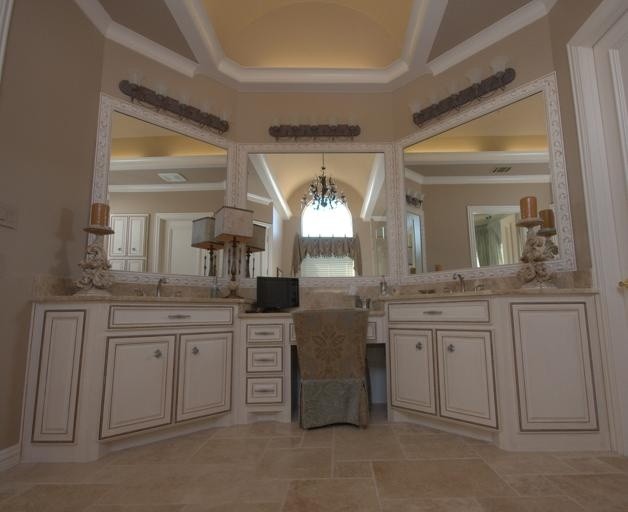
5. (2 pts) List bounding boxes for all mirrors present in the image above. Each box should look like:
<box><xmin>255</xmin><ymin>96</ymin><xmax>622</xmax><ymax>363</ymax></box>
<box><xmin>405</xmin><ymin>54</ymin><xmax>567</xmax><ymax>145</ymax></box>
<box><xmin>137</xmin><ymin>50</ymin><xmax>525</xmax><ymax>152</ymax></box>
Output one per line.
<box><xmin>466</xmin><ymin>205</ymin><xmax>528</xmax><ymax>268</ymax></box>
<box><xmin>237</xmin><ymin>142</ymin><xmax>398</xmax><ymax>289</ymax></box>
<box><xmin>406</xmin><ymin>205</ymin><xmax>427</xmax><ymax>275</ymax></box>
<box><xmin>85</xmin><ymin>91</ymin><xmax>237</xmax><ymax>298</ymax></box>
<box><xmin>399</xmin><ymin>71</ymin><xmax>577</xmax><ymax>286</ymax></box>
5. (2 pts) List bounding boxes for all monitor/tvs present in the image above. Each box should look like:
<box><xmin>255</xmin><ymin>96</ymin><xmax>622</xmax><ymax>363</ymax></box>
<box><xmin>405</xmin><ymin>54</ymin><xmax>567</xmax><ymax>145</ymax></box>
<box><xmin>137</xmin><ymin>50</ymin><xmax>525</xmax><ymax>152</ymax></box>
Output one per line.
<box><xmin>256</xmin><ymin>276</ymin><xmax>299</xmax><ymax>313</ymax></box>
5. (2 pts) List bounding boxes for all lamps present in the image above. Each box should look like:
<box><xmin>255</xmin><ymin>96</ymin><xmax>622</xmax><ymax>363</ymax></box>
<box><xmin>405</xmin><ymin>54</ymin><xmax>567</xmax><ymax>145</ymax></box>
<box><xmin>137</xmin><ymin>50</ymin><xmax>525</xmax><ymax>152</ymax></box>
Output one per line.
<box><xmin>215</xmin><ymin>206</ymin><xmax>254</xmax><ymax>298</ymax></box>
<box><xmin>191</xmin><ymin>218</ymin><xmax>224</xmax><ymax>277</ymax></box>
<box><xmin>248</xmin><ymin>224</ymin><xmax>265</xmax><ymax>277</ymax></box>
<box><xmin>300</xmin><ymin>153</ymin><xmax>347</xmax><ymax>213</ymax></box>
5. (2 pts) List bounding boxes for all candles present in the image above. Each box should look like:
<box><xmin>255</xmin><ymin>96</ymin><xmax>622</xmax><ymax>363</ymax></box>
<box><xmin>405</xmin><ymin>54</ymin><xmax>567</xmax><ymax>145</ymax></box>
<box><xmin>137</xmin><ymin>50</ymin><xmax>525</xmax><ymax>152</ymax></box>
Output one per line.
<box><xmin>520</xmin><ymin>197</ymin><xmax>538</xmax><ymax>218</ymax></box>
<box><xmin>540</xmin><ymin>210</ymin><xmax>556</xmax><ymax>229</ymax></box>
<box><xmin>91</xmin><ymin>203</ymin><xmax>109</xmax><ymax>226</ymax></box>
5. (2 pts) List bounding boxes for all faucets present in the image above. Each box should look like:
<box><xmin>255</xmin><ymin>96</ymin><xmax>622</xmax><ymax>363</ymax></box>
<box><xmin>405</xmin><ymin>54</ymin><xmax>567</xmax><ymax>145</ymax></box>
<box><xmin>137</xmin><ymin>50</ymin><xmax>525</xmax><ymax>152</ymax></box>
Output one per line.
<box><xmin>154</xmin><ymin>275</ymin><xmax>169</xmax><ymax>296</ymax></box>
<box><xmin>452</xmin><ymin>271</ymin><xmax>465</xmax><ymax>292</ymax></box>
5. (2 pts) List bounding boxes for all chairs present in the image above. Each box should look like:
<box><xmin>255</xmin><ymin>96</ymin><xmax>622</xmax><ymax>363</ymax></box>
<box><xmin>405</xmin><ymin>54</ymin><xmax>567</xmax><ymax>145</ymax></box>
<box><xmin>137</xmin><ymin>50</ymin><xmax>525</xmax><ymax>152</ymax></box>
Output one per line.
<box><xmin>288</xmin><ymin>310</ymin><xmax>372</xmax><ymax>428</ymax></box>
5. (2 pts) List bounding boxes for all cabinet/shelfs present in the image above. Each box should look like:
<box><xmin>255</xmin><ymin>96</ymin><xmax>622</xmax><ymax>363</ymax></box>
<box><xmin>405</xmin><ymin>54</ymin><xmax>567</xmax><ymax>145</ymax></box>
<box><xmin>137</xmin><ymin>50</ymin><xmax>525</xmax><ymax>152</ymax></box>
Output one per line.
<box><xmin>104</xmin><ymin>213</ymin><xmax>150</xmax><ymax>272</ymax></box>
<box><xmin>19</xmin><ymin>304</ymin><xmax>241</xmax><ymax>464</ymax></box>
<box><xmin>240</xmin><ymin>319</ymin><xmax>384</xmax><ymax>424</ymax></box>
<box><xmin>384</xmin><ymin>294</ymin><xmax>612</xmax><ymax>455</ymax></box>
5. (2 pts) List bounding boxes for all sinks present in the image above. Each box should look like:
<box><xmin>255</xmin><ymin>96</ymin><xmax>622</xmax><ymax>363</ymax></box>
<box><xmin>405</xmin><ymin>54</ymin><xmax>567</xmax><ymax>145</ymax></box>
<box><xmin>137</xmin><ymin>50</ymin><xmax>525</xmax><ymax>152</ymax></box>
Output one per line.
<box><xmin>438</xmin><ymin>292</ymin><xmax>488</xmax><ymax>297</ymax></box>
